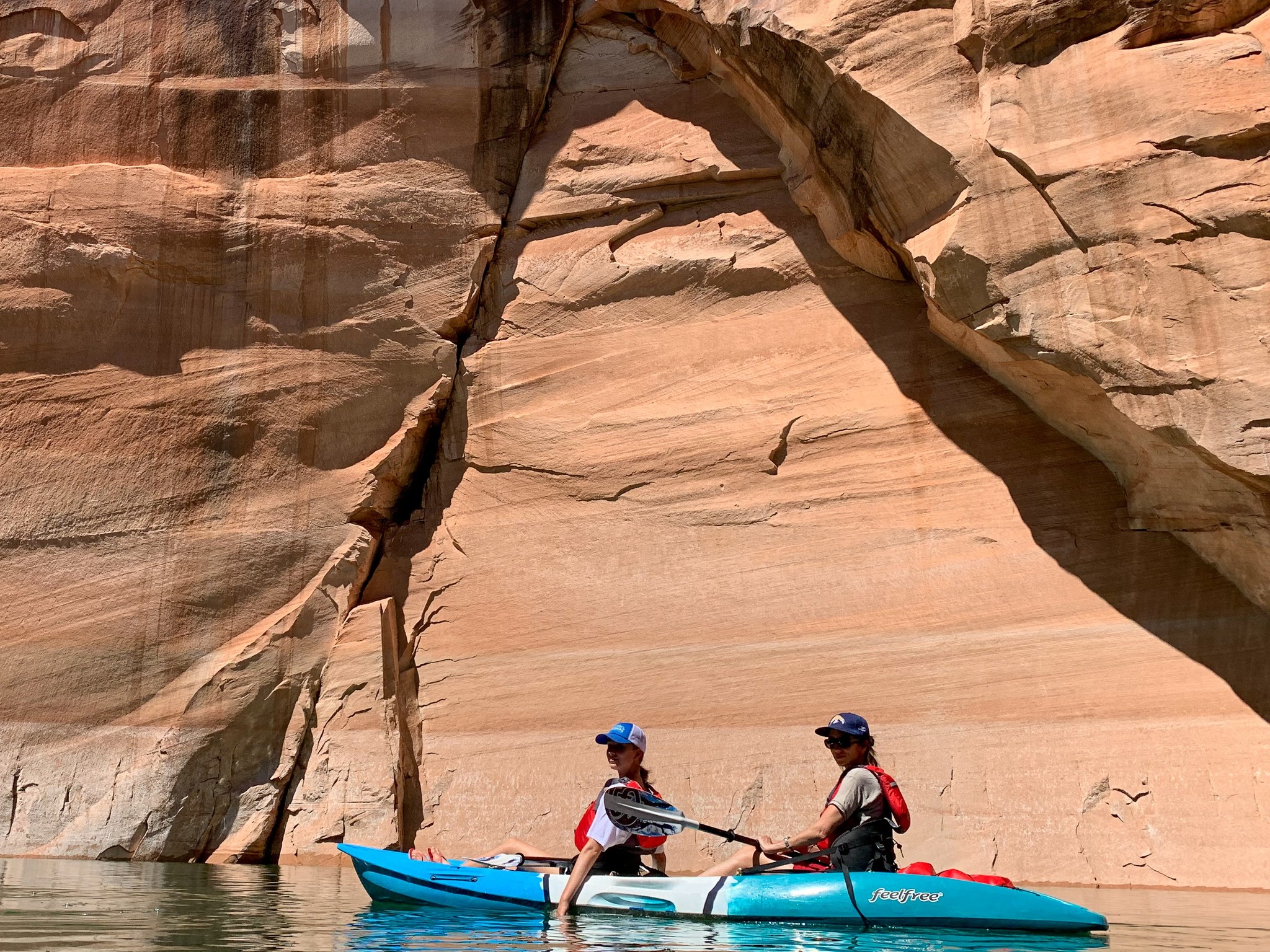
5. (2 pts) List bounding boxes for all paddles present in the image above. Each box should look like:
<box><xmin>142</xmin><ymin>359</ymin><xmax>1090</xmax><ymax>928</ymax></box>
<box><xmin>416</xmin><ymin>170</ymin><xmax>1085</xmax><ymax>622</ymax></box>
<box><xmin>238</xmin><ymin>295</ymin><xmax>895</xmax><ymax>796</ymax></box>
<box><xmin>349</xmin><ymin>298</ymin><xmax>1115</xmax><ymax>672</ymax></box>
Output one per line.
<box><xmin>603</xmin><ymin>785</ymin><xmax>825</xmax><ymax>862</ymax></box>
<box><xmin>461</xmin><ymin>850</ymin><xmax>571</xmax><ymax>871</ymax></box>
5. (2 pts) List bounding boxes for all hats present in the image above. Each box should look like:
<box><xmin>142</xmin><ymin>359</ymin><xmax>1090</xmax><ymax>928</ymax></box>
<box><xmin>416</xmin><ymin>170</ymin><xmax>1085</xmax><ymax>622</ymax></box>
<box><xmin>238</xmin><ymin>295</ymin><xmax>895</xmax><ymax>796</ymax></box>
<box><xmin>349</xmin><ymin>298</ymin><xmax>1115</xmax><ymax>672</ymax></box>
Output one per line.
<box><xmin>815</xmin><ymin>712</ymin><xmax>870</xmax><ymax>740</ymax></box>
<box><xmin>595</xmin><ymin>722</ymin><xmax>646</xmax><ymax>754</ymax></box>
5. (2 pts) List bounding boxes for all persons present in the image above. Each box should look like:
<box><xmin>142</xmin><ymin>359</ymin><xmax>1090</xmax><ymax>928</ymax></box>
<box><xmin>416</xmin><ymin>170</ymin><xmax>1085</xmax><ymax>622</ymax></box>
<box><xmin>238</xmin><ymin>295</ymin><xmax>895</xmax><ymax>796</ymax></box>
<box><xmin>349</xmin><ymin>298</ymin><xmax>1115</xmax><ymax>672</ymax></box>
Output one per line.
<box><xmin>695</xmin><ymin>712</ymin><xmax>897</xmax><ymax>877</ymax></box>
<box><xmin>408</xmin><ymin>722</ymin><xmax>668</xmax><ymax>918</ymax></box>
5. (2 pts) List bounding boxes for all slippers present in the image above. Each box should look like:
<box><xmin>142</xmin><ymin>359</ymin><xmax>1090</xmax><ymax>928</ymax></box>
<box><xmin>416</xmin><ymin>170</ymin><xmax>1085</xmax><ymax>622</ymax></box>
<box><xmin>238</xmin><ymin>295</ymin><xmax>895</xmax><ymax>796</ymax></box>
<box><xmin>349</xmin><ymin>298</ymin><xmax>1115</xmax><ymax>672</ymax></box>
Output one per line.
<box><xmin>409</xmin><ymin>848</ymin><xmax>426</xmax><ymax>861</ymax></box>
<box><xmin>427</xmin><ymin>847</ymin><xmax>448</xmax><ymax>864</ymax></box>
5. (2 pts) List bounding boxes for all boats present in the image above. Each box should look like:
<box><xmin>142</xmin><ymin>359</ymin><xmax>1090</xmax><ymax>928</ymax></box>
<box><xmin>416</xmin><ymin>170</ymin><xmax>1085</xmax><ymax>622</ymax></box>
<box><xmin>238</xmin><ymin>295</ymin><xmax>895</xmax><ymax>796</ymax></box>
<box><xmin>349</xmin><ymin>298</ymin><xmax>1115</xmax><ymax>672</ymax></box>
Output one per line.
<box><xmin>333</xmin><ymin>840</ymin><xmax>1110</xmax><ymax>936</ymax></box>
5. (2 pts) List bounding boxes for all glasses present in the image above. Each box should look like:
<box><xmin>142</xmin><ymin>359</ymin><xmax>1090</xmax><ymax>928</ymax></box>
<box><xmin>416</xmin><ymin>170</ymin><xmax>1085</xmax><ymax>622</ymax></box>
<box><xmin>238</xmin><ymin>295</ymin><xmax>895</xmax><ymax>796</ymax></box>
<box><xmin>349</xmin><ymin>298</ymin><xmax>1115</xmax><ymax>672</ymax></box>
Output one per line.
<box><xmin>824</xmin><ymin>735</ymin><xmax>867</xmax><ymax>749</ymax></box>
